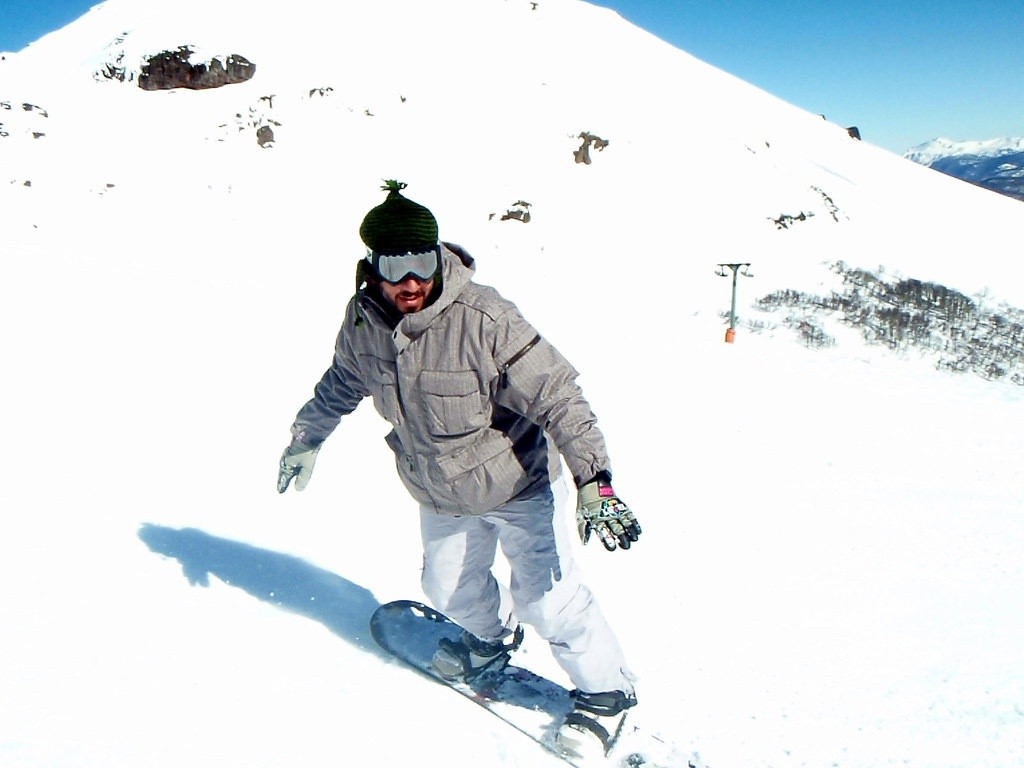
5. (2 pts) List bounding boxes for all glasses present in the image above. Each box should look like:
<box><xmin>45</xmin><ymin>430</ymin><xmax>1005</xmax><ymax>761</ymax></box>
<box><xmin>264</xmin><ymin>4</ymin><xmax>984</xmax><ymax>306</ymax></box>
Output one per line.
<box><xmin>364</xmin><ymin>245</ymin><xmax>440</xmax><ymax>285</ymax></box>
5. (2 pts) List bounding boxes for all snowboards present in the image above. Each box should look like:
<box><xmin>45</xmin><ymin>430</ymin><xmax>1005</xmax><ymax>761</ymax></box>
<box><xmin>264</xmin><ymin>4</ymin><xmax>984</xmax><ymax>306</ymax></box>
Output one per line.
<box><xmin>371</xmin><ymin>599</ymin><xmax>710</xmax><ymax>768</ymax></box>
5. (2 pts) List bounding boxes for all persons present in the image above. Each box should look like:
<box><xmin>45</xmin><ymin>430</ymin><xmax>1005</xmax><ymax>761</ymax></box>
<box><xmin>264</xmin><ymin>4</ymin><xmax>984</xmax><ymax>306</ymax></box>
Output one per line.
<box><xmin>276</xmin><ymin>180</ymin><xmax>642</xmax><ymax>766</ymax></box>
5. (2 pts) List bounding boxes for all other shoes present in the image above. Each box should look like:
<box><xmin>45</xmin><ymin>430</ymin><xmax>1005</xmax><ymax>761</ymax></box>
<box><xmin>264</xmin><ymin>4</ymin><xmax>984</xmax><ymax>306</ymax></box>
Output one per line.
<box><xmin>434</xmin><ymin>630</ymin><xmax>524</xmax><ymax>682</ymax></box>
<box><xmin>557</xmin><ymin>687</ymin><xmax>638</xmax><ymax>760</ymax></box>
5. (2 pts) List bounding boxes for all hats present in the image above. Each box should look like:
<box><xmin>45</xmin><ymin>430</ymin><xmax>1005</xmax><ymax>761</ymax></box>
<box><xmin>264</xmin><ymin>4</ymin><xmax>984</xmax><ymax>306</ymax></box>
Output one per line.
<box><xmin>354</xmin><ymin>180</ymin><xmax>444</xmax><ymax>312</ymax></box>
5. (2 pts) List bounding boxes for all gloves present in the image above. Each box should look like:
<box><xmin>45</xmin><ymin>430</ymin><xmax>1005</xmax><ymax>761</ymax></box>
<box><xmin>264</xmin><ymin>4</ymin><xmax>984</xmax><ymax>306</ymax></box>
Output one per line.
<box><xmin>276</xmin><ymin>426</ymin><xmax>325</xmax><ymax>493</ymax></box>
<box><xmin>575</xmin><ymin>472</ymin><xmax>642</xmax><ymax>553</ymax></box>
